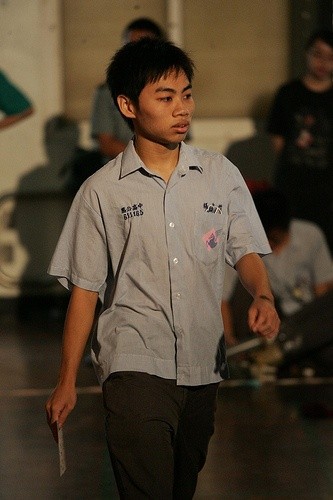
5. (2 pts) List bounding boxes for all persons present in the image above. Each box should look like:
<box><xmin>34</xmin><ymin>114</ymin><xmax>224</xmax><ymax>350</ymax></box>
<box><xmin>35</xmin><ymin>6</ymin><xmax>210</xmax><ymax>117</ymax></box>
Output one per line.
<box><xmin>46</xmin><ymin>37</ymin><xmax>280</xmax><ymax>500</ymax></box>
<box><xmin>267</xmin><ymin>29</ymin><xmax>333</xmax><ymax>258</ymax></box>
<box><xmin>91</xmin><ymin>18</ymin><xmax>169</xmax><ymax>166</ymax></box>
<box><xmin>221</xmin><ymin>188</ymin><xmax>333</xmax><ymax>379</ymax></box>
